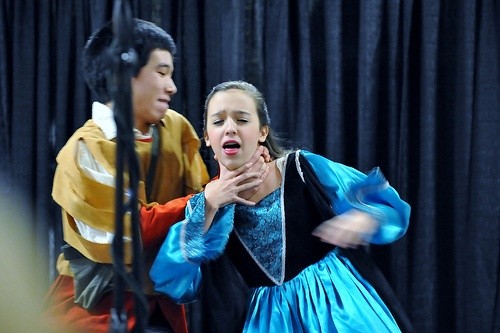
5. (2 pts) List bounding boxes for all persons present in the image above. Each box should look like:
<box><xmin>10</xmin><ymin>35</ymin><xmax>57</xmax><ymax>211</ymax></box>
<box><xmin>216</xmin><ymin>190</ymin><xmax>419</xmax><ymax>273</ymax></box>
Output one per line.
<box><xmin>37</xmin><ymin>15</ymin><xmax>264</xmax><ymax>333</ymax></box>
<box><xmin>147</xmin><ymin>79</ymin><xmax>422</xmax><ymax>333</ymax></box>
